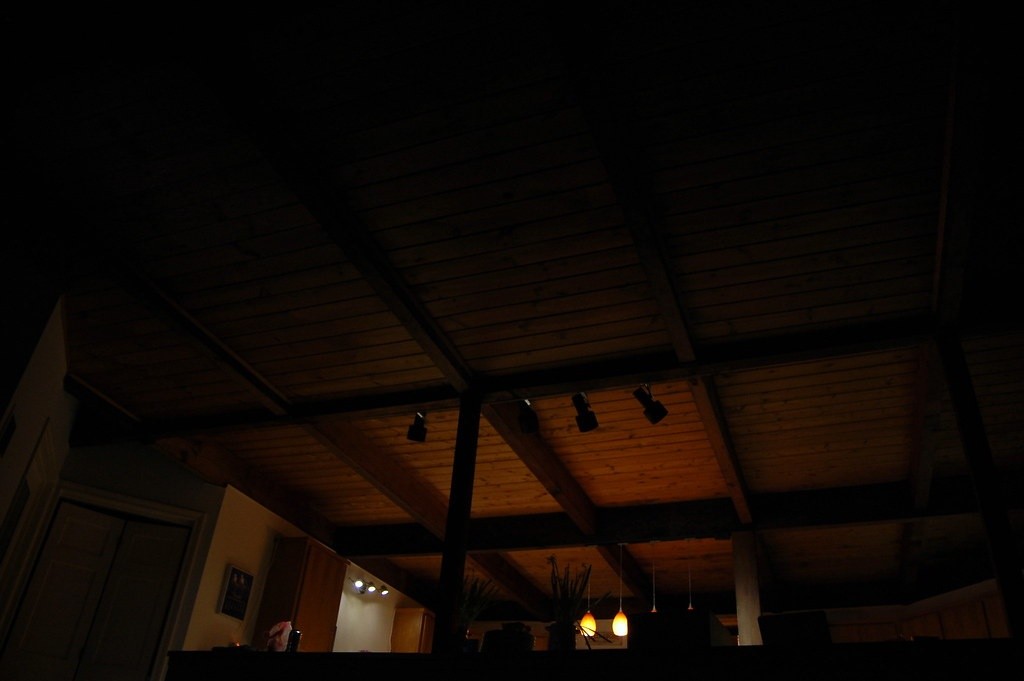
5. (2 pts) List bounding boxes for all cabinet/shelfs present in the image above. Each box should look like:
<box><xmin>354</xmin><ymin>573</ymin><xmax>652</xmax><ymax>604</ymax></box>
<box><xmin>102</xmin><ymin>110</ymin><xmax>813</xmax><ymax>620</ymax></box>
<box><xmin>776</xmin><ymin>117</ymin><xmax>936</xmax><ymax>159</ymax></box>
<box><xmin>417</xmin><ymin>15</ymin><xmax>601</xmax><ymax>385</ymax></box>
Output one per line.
<box><xmin>710</xmin><ymin>579</ymin><xmax>1011</xmax><ymax>647</ymax></box>
<box><xmin>0</xmin><ymin>299</ymin><xmax>74</xmax><ymax>640</ymax></box>
<box><xmin>252</xmin><ymin>533</ymin><xmax>351</xmax><ymax>650</ymax></box>
<box><xmin>390</xmin><ymin>607</ymin><xmax>437</xmax><ymax>652</ymax></box>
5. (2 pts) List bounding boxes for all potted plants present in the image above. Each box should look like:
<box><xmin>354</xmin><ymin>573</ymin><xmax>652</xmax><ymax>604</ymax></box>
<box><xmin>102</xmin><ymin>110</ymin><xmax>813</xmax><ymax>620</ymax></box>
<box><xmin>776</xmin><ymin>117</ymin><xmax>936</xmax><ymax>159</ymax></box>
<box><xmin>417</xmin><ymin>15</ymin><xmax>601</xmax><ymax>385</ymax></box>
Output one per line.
<box><xmin>519</xmin><ymin>554</ymin><xmax>592</xmax><ymax>650</ymax></box>
<box><xmin>439</xmin><ymin>571</ymin><xmax>499</xmax><ymax>651</ymax></box>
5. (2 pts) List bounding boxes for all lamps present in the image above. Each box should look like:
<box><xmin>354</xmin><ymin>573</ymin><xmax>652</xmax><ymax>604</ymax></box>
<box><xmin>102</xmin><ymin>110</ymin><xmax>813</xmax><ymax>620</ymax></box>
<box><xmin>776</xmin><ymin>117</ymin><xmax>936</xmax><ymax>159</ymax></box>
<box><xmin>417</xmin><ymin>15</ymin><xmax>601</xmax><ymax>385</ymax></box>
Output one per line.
<box><xmin>569</xmin><ymin>390</ymin><xmax>598</xmax><ymax>432</ymax></box>
<box><xmin>611</xmin><ymin>542</ymin><xmax>629</xmax><ymax>636</ymax></box>
<box><xmin>580</xmin><ymin>571</ymin><xmax>597</xmax><ymax>637</ymax></box>
<box><xmin>347</xmin><ymin>577</ymin><xmax>394</xmax><ymax>597</ymax></box>
<box><xmin>409</xmin><ymin>405</ymin><xmax>430</xmax><ymax>443</ymax></box>
<box><xmin>630</xmin><ymin>386</ymin><xmax>670</xmax><ymax>424</ymax></box>
<box><xmin>515</xmin><ymin>396</ymin><xmax>537</xmax><ymax>436</ymax></box>
